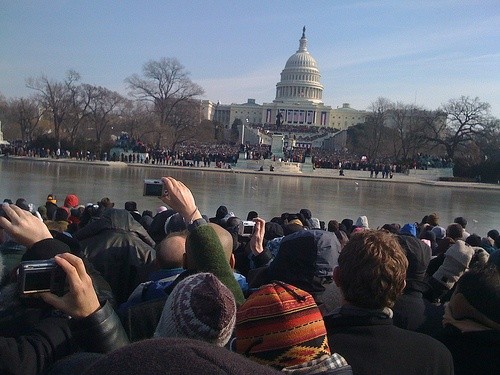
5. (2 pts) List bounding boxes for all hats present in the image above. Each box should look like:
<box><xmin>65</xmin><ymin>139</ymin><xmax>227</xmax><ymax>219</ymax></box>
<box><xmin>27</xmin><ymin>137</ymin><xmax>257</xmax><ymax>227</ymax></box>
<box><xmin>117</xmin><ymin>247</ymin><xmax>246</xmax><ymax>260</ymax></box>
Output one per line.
<box><xmin>97</xmin><ymin>197</ymin><xmax>114</xmax><ymax>208</ymax></box>
<box><xmin>236</xmin><ymin>280</ymin><xmax>331</xmax><ymax>368</ymax></box>
<box><xmin>437</xmin><ymin>236</ymin><xmax>455</xmax><ymax>250</ymax></box>
<box><xmin>216</xmin><ymin>206</ymin><xmax>229</xmax><ymax>219</ymax></box>
<box><xmin>399</xmin><ymin>223</ymin><xmax>416</xmax><ymax>236</ymax></box>
<box><xmin>309</xmin><ymin>218</ymin><xmax>320</xmax><ymax>229</ymax></box>
<box><xmin>155</xmin><ymin>206</ymin><xmax>168</xmax><ymax>213</ymax></box>
<box><xmin>265</xmin><ymin>221</ymin><xmax>284</xmax><ymax>240</ymax></box>
<box><xmin>396</xmin><ymin>234</ymin><xmax>431</xmax><ymax>281</ymax></box>
<box><xmin>454</xmin><ymin>217</ymin><xmax>468</xmax><ymax>228</ymax></box>
<box><xmin>124</xmin><ymin>201</ymin><xmax>139</xmax><ymax>211</ymax></box>
<box><xmin>154</xmin><ymin>272</ymin><xmax>237</xmax><ymax>350</ymax></box>
<box><xmin>488</xmin><ymin>229</ymin><xmax>499</xmax><ymax>242</ymax></box>
<box><xmin>425</xmin><ymin>213</ymin><xmax>440</xmax><ymax>225</ymax></box>
<box><xmin>351</xmin><ymin>228</ymin><xmax>362</xmax><ymax>234</ymax></box>
<box><xmin>431</xmin><ymin>226</ymin><xmax>446</xmax><ymax>239</ymax></box>
<box><xmin>47</xmin><ymin>194</ymin><xmax>57</xmax><ymax>200</ymax></box>
<box><xmin>141</xmin><ymin>211</ymin><xmax>154</xmax><ymax>218</ymax></box>
<box><xmin>328</xmin><ymin>220</ymin><xmax>339</xmax><ymax>232</ymax></box>
<box><xmin>64</xmin><ymin>194</ymin><xmax>79</xmax><ymax>208</ymax></box>
<box><xmin>140</xmin><ymin>216</ymin><xmax>153</xmax><ymax>228</ymax></box>
<box><xmin>356</xmin><ymin>216</ymin><xmax>368</xmax><ymax>227</ymax></box>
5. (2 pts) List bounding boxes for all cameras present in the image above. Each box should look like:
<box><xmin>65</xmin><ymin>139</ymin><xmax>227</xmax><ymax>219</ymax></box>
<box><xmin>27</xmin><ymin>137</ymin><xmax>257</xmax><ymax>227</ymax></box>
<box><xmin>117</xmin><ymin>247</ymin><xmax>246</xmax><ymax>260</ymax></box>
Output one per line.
<box><xmin>237</xmin><ymin>221</ymin><xmax>257</xmax><ymax>236</ymax></box>
<box><xmin>16</xmin><ymin>260</ymin><xmax>66</xmax><ymax>294</ymax></box>
<box><xmin>143</xmin><ymin>179</ymin><xmax>169</xmax><ymax>197</ymax></box>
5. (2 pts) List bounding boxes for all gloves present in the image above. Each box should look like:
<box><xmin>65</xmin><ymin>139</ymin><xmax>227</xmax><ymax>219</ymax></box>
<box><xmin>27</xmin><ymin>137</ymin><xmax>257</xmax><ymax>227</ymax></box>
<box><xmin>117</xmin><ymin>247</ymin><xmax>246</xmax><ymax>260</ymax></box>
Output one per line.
<box><xmin>433</xmin><ymin>240</ymin><xmax>474</xmax><ymax>289</ymax></box>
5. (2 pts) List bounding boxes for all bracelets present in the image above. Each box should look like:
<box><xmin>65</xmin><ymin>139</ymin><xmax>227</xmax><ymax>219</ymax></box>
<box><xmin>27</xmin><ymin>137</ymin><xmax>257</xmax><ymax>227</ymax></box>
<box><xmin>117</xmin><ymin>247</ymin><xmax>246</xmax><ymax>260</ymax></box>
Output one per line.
<box><xmin>185</xmin><ymin>208</ymin><xmax>197</xmax><ymax>223</ymax></box>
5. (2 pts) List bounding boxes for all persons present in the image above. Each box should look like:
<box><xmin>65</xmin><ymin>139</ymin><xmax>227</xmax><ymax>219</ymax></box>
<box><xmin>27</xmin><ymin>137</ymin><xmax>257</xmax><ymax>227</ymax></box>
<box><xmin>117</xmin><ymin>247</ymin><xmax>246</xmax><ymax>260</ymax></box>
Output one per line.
<box><xmin>1</xmin><ymin>176</ymin><xmax>499</xmax><ymax>374</ymax></box>
<box><xmin>1</xmin><ymin>135</ymin><xmax>458</xmax><ymax>179</ymax></box>
<box><xmin>276</xmin><ymin>108</ymin><xmax>284</xmax><ymax>133</ymax></box>
